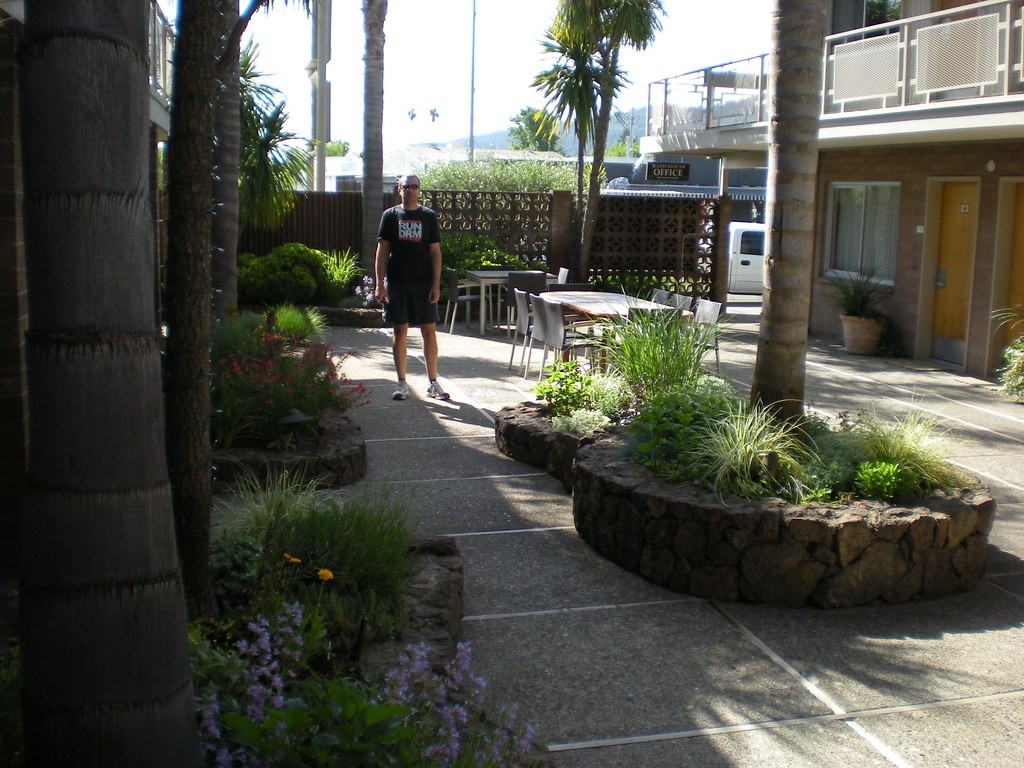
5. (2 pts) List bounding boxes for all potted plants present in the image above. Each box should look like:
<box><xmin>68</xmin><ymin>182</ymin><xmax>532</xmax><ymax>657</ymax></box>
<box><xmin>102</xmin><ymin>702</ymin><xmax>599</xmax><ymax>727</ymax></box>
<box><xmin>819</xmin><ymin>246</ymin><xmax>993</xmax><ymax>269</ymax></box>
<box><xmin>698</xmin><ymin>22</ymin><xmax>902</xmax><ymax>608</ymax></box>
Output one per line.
<box><xmin>827</xmin><ymin>264</ymin><xmax>888</xmax><ymax>355</ymax></box>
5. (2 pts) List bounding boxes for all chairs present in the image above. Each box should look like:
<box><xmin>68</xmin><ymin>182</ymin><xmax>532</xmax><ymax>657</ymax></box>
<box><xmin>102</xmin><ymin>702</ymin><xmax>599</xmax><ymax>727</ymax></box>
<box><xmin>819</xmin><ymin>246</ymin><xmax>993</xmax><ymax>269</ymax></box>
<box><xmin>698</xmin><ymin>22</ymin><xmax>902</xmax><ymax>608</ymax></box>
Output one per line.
<box><xmin>443</xmin><ymin>267</ymin><xmax>722</xmax><ymax>381</ymax></box>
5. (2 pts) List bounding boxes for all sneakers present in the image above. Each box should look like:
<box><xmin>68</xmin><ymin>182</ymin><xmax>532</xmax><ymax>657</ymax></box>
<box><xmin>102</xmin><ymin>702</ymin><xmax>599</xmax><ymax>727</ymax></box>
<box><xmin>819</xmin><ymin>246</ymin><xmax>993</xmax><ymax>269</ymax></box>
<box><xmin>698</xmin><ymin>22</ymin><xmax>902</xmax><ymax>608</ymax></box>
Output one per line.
<box><xmin>393</xmin><ymin>382</ymin><xmax>409</xmax><ymax>399</ymax></box>
<box><xmin>426</xmin><ymin>380</ymin><xmax>449</xmax><ymax>399</ymax></box>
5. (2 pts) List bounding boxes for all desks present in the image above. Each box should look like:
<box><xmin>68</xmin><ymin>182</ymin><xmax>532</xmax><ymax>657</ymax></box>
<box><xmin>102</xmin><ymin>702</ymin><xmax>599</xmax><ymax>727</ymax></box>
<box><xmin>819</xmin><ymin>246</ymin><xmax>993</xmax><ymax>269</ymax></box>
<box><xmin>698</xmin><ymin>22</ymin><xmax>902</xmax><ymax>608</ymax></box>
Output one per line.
<box><xmin>539</xmin><ymin>291</ymin><xmax>694</xmax><ymax>374</ymax></box>
<box><xmin>467</xmin><ymin>270</ymin><xmax>558</xmax><ymax>336</ymax></box>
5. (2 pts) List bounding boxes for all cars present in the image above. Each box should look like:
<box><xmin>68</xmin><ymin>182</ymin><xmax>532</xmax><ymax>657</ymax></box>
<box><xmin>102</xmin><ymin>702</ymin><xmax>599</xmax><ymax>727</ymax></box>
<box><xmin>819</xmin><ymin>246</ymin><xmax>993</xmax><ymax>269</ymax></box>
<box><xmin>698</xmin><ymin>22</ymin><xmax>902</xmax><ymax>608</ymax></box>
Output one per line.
<box><xmin>695</xmin><ymin>221</ymin><xmax>769</xmax><ymax>296</ymax></box>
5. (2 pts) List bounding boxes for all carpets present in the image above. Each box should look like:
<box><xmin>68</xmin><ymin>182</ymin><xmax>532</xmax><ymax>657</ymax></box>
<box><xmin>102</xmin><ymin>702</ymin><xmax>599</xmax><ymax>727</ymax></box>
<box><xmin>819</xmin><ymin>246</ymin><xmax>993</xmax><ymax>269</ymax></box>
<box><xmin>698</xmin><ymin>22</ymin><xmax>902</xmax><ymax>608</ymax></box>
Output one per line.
<box><xmin>885</xmin><ymin>359</ymin><xmax>955</xmax><ymax>371</ymax></box>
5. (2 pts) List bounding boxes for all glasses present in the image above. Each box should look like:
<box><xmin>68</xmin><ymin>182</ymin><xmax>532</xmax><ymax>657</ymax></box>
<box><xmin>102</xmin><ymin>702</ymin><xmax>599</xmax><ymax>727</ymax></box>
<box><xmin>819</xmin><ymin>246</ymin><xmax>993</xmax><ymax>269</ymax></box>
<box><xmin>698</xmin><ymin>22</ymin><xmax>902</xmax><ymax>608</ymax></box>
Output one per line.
<box><xmin>399</xmin><ymin>184</ymin><xmax>419</xmax><ymax>190</ymax></box>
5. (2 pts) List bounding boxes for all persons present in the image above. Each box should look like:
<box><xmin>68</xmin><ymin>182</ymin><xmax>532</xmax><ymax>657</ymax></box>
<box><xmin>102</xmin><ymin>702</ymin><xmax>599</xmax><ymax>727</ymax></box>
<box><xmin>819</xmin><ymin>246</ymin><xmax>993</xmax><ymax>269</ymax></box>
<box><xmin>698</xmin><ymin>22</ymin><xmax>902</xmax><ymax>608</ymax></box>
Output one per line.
<box><xmin>374</xmin><ymin>174</ymin><xmax>451</xmax><ymax>399</ymax></box>
